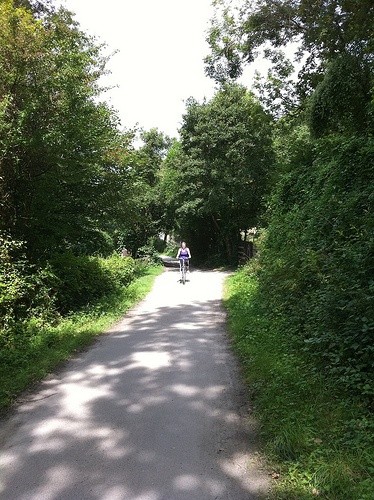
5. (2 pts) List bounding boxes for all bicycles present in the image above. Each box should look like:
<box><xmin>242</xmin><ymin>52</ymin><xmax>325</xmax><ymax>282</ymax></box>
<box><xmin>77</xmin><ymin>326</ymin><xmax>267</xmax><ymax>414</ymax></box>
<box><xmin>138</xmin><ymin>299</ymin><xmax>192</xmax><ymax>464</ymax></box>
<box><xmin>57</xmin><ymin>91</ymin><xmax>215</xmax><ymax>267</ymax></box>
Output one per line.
<box><xmin>179</xmin><ymin>258</ymin><xmax>190</xmax><ymax>284</ymax></box>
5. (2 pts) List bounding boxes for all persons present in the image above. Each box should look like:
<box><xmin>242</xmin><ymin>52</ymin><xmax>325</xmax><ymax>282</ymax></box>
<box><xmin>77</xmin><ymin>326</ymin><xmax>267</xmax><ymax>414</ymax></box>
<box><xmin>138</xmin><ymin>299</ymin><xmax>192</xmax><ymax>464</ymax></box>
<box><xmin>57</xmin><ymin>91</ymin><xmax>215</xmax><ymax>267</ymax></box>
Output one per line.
<box><xmin>175</xmin><ymin>241</ymin><xmax>192</xmax><ymax>274</ymax></box>
